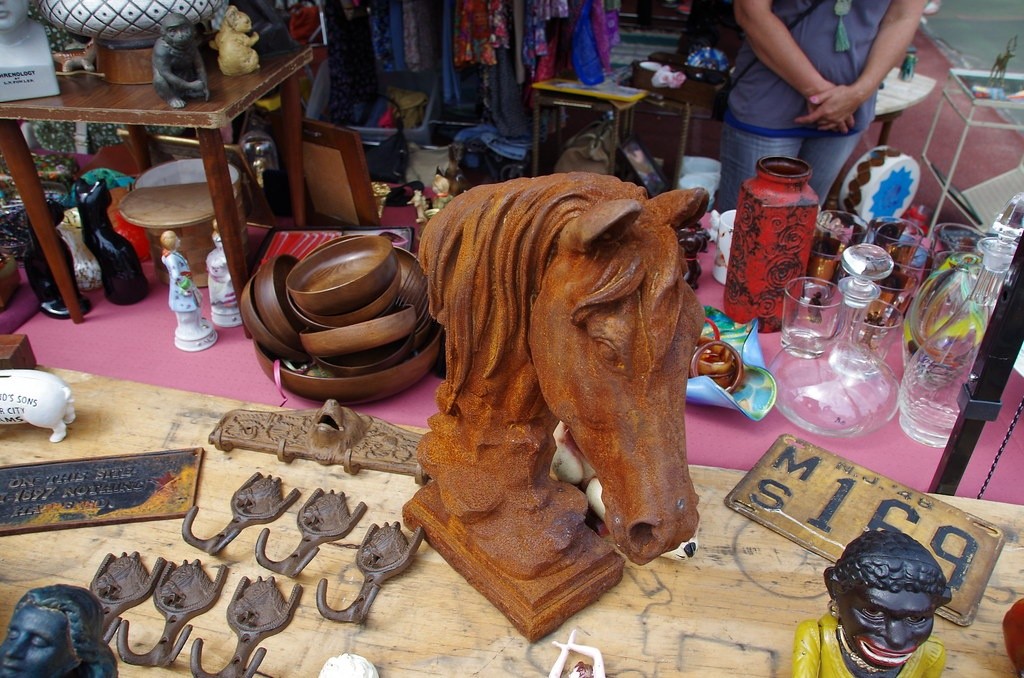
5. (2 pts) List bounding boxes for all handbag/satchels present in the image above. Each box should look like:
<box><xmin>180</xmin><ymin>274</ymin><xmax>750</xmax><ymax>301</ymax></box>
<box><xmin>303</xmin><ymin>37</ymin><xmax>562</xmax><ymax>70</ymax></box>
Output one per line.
<box><xmin>554</xmin><ymin>111</ymin><xmax>620</xmax><ymax>175</ymax></box>
<box><xmin>335</xmin><ymin>96</ymin><xmax>410</xmax><ymax>184</ymax></box>
<box><xmin>711</xmin><ymin>84</ymin><xmax>730</xmax><ymax>124</ymax></box>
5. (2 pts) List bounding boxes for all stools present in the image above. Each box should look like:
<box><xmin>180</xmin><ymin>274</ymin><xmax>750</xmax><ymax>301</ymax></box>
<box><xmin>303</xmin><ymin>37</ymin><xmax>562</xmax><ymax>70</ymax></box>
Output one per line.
<box><xmin>533</xmin><ymin>91</ymin><xmax>690</xmax><ymax>177</ymax></box>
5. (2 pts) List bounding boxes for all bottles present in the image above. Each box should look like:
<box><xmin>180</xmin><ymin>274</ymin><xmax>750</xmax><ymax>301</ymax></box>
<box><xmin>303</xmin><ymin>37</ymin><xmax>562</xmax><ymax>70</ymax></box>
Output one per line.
<box><xmin>766</xmin><ymin>298</ymin><xmax>899</xmax><ymax>438</ymax></box>
<box><xmin>897</xmin><ymin>193</ymin><xmax>1024</xmax><ymax>451</ymax></box>
<box><xmin>722</xmin><ymin>156</ymin><xmax>819</xmax><ymax>332</ymax></box>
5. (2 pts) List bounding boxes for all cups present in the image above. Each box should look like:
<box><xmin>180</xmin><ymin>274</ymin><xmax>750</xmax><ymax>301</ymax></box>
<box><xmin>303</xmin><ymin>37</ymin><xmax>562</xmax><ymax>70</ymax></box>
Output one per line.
<box><xmin>780</xmin><ymin>210</ymin><xmax>984</xmax><ymax>359</ymax></box>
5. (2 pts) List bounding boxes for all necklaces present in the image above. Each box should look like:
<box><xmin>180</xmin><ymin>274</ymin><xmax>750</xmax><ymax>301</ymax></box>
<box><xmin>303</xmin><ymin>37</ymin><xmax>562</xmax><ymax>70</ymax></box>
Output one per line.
<box><xmin>833</xmin><ymin>0</ymin><xmax>852</xmax><ymax>52</ymax></box>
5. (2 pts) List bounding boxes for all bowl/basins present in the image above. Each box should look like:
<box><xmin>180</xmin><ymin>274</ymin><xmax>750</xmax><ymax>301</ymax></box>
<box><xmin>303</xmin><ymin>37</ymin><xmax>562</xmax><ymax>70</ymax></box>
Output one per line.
<box><xmin>241</xmin><ymin>236</ymin><xmax>444</xmax><ymax>405</ymax></box>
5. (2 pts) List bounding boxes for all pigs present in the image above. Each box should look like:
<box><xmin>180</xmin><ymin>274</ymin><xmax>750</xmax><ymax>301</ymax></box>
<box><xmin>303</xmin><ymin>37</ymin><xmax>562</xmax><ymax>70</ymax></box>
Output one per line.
<box><xmin>0</xmin><ymin>370</ymin><xmax>77</xmax><ymax>443</ymax></box>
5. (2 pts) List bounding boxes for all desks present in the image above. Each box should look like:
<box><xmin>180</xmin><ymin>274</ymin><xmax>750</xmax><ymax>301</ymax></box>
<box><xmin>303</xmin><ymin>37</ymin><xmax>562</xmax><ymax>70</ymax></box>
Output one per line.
<box><xmin>0</xmin><ymin>364</ymin><xmax>1024</xmax><ymax>678</ymax></box>
<box><xmin>0</xmin><ymin>46</ymin><xmax>314</xmax><ymax>340</ymax></box>
<box><xmin>873</xmin><ymin>67</ymin><xmax>937</xmax><ymax>146</ymax></box>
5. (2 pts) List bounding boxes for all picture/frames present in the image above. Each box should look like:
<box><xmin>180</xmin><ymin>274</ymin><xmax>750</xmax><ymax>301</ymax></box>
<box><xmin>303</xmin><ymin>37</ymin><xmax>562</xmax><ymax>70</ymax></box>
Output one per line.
<box><xmin>302</xmin><ymin>118</ymin><xmax>381</xmax><ymax>227</ymax></box>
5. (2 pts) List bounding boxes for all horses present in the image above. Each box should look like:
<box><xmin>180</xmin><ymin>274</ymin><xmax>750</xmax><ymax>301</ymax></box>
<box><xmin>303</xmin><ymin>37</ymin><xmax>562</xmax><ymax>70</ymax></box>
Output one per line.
<box><xmin>417</xmin><ymin>173</ymin><xmax>709</xmax><ymax>575</ymax></box>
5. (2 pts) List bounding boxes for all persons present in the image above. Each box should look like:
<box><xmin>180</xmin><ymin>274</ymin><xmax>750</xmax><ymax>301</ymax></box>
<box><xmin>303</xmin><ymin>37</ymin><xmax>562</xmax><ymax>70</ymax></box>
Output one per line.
<box><xmin>206</xmin><ymin>219</ymin><xmax>245</xmax><ymax>328</ymax></box>
<box><xmin>0</xmin><ymin>583</ymin><xmax>119</xmax><ymax>678</ymax></box>
<box><xmin>714</xmin><ymin>0</ymin><xmax>928</xmax><ymax>223</ymax></box>
<box><xmin>0</xmin><ymin>0</ymin><xmax>61</xmax><ymax>103</ymax></box>
<box><xmin>160</xmin><ymin>229</ymin><xmax>219</xmax><ymax>352</ymax></box>
<box><xmin>791</xmin><ymin>529</ymin><xmax>952</xmax><ymax>678</ymax></box>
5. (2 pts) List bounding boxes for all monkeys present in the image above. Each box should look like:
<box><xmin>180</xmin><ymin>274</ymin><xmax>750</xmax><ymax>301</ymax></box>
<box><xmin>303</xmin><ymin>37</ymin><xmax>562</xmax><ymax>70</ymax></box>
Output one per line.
<box><xmin>151</xmin><ymin>13</ymin><xmax>210</xmax><ymax>108</ymax></box>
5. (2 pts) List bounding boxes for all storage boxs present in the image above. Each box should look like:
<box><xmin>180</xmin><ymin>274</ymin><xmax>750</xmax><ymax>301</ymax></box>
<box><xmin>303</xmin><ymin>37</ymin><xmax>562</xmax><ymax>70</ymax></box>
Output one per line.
<box><xmin>631</xmin><ymin>0</ymin><xmax>744</xmax><ymax>107</ymax></box>
